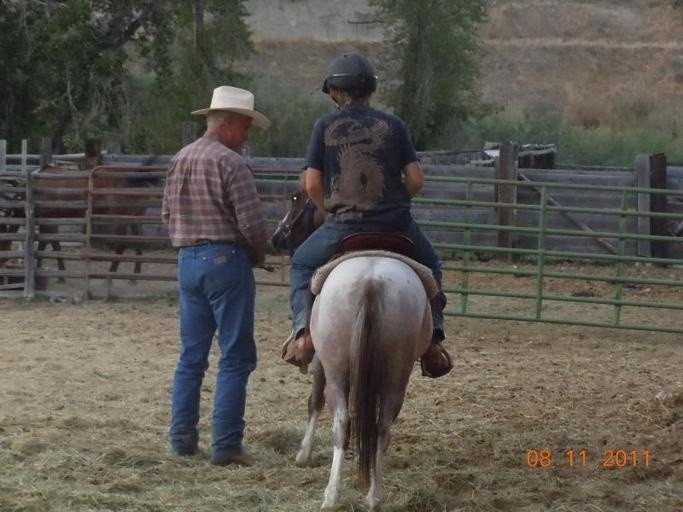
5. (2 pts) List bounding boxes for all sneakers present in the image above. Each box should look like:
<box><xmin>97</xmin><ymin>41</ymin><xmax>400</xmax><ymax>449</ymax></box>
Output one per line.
<box><xmin>171</xmin><ymin>439</ymin><xmax>199</xmax><ymax>457</ymax></box>
<box><xmin>419</xmin><ymin>341</ymin><xmax>450</xmax><ymax>378</ymax></box>
<box><xmin>283</xmin><ymin>331</ymin><xmax>315</xmax><ymax>369</ymax></box>
<box><xmin>211</xmin><ymin>450</ymin><xmax>256</xmax><ymax>467</ymax></box>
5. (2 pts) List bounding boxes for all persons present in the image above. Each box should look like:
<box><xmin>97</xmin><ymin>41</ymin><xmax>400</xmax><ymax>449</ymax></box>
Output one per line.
<box><xmin>281</xmin><ymin>52</ymin><xmax>453</xmax><ymax>379</ymax></box>
<box><xmin>160</xmin><ymin>85</ymin><xmax>271</xmax><ymax>468</ymax></box>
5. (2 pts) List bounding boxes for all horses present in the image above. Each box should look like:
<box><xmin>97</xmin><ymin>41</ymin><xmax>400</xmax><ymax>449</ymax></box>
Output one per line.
<box><xmin>264</xmin><ymin>184</ymin><xmax>436</xmax><ymax>512</ymax></box>
<box><xmin>0</xmin><ymin>165</ymin><xmax>164</xmax><ymax>287</ymax></box>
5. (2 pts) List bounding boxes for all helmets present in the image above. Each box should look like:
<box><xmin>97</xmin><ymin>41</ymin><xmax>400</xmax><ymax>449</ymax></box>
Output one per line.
<box><xmin>322</xmin><ymin>52</ymin><xmax>378</xmax><ymax>96</ymax></box>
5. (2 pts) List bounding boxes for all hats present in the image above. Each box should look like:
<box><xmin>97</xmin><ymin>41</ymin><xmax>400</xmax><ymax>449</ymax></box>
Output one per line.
<box><xmin>189</xmin><ymin>85</ymin><xmax>271</xmax><ymax>131</ymax></box>
